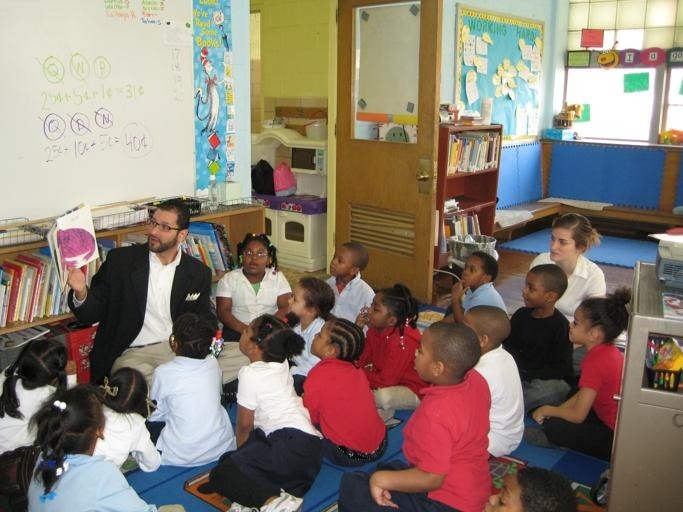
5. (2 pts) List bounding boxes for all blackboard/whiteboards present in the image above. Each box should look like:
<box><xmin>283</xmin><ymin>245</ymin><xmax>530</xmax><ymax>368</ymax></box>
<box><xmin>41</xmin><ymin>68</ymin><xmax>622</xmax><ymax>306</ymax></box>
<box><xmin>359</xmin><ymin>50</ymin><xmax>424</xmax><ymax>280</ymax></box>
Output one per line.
<box><xmin>1</xmin><ymin>0</ymin><xmax>194</xmax><ymax>226</ymax></box>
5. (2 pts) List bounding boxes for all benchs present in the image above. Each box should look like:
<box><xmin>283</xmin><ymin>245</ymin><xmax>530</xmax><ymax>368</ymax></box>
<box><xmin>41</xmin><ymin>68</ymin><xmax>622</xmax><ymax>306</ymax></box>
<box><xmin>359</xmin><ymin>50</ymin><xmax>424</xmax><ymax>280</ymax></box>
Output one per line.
<box><xmin>492</xmin><ymin>190</ymin><xmax>682</xmax><ymax>241</ymax></box>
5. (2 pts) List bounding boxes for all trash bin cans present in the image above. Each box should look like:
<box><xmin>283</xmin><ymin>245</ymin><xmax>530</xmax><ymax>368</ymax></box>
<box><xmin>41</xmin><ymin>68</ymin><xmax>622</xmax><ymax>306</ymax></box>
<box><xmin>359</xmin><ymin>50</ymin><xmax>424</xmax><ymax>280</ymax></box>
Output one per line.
<box><xmin>448</xmin><ymin>234</ymin><xmax>498</xmax><ymax>287</ymax></box>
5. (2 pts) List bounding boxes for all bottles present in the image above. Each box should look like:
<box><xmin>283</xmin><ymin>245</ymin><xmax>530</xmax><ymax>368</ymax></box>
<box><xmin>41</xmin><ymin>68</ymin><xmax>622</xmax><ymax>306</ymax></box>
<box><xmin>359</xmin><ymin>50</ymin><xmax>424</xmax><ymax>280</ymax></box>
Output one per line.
<box><xmin>207</xmin><ymin>175</ymin><xmax>219</xmax><ymax>210</ymax></box>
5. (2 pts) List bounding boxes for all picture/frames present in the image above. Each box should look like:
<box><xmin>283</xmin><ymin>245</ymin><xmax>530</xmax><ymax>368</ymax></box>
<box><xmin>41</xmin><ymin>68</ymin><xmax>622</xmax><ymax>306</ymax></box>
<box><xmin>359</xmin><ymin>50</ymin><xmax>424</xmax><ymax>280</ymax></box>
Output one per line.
<box><xmin>565</xmin><ymin>49</ymin><xmax>591</xmax><ymax>68</ymax></box>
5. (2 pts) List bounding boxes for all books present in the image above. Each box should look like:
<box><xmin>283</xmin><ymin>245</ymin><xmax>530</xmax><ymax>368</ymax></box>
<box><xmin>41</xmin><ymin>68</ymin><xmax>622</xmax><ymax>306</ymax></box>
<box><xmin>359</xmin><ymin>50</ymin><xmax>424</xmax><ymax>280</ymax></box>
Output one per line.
<box><xmin>95</xmin><ymin>234</ymin><xmax>149</xmax><ymax>264</ymax></box>
<box><xmin>1</xmin><ymin>254</ymin><xmax>66</xmax><ymax>328</ymax></box>
<box><xmin>441</xmin><ymin>214</ymin><xmax>480</xmax><ymax>254</ymax></box>
<box><xmin>433</xmin><ymin>126</ymin><xmax>501</xmax><ymax>256</ymax></box>
<box><xmin>39</xmin><ymin>245</ymin><xmax>112</xmax><ymax>317</ymax></box>
<box><xmin>443</xmin><ymin>199</ymin><xmax>463</xmax><ymax>215</ymax></box>
<box><xmin>45</xmin><ymin>203</ymin><xmax>100</xmax><ymax>293</ymax></box>
<box><xmin>178</xmin><ymin>221</ymin><xmax>234</xmax><ymax>279</ymax></box>
<box><xmin>2</xmin><ymin>326</ymin><xmax>50</xmax><ymax>351</ymax></box>
<box><xmin>434</xmin><ymin>209</ymin><xmax>439</xmax><ymax>247</ymax></box>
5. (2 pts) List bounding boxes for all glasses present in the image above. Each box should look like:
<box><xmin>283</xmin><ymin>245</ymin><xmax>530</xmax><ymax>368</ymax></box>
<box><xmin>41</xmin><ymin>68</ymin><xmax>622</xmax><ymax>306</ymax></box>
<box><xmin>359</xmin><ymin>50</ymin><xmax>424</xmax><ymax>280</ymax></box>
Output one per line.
<box><xmin>148</xmin><ymin>219</ymin><xmax>182</xmax><ymax>232</ymax></box>
<box><xmin>245</xmin><ymin>250</ymin><xmax>267</xmax><ymax>258</ymax></box>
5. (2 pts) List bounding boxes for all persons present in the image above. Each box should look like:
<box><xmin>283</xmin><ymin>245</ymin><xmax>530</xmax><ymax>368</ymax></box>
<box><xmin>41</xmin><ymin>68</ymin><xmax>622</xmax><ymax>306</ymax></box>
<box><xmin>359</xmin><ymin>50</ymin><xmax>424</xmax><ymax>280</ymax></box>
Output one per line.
<box><xmin>0</xmin><ymin>339</ymin><xmax>68</xmax><ymax>511</ymax></box>
<box><xmin>144</xmin><ymin>307</ymin><xmax>236</xmax><ymax>466</ymax></box>
<box><xmin>286</xmin><ymin>277</ymin><xmax>335</xmax><ymax>396</ymax></box>
<box><xmin>27</xmin><ymin>388</ymin><xmax>157</xmax><ymax>511</ymax></box>
<box><xmin>300</xmin><ymin>241</ymin><xmax>633</xmax><ymax>512</ymax></box>
<box><xmin>209</xmin><ymin>314</ymin><xmax>324</xmax><ymax>511</ymax></box>
<box><xmin>215</xmin><ymin>232</ymin><xmax>292</xmax><ymax>344</ymax></box>
<box><xmin>64</xmin><ymin>199</ymin><xmax>254</xmax><ymax>444</ymax></box>
<box><xmin>529</xmin><ymin>213</ymin><xmax>607</xmax><ymax>325</ymax></box>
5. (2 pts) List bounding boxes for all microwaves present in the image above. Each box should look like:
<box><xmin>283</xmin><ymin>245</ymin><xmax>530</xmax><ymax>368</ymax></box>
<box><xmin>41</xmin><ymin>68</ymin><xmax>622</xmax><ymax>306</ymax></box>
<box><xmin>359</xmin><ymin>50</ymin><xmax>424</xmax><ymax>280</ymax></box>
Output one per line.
<box><xmin>286</xmin><ymin>145</ymin><xmax>327</xmax><ymax>176</ymax></box>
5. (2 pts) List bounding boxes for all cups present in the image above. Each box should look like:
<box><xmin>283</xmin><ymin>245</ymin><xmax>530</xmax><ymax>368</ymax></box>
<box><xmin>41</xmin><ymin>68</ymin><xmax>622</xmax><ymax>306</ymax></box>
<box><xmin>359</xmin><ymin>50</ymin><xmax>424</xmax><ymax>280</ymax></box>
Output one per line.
<box><xmin>481</xmin><ymin>97</ymin><xmax>493</xmax><ymax>125</ymax></box>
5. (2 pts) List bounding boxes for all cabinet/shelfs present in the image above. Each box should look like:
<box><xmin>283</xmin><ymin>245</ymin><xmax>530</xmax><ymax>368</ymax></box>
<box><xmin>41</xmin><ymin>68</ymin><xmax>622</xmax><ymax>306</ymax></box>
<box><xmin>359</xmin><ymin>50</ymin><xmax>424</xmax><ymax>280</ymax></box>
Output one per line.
<box><xmin>603</xmin><ymin>257</ymin><xmax>682</xmax><ymax>512</ymax></box>
<box><xmin>432</xmin><ymin>122</ymin><xmax>503</xmax><ymax>273</ymax></box>
<box><xmin>263</xmin><ymin>208</ymin><xmax>327</xmax><ymax>273</ymax></box>
<box><xmin>0</xmin><ymin>205</ymin><xmax>266</xmax><ymax>338</ymax></box>
